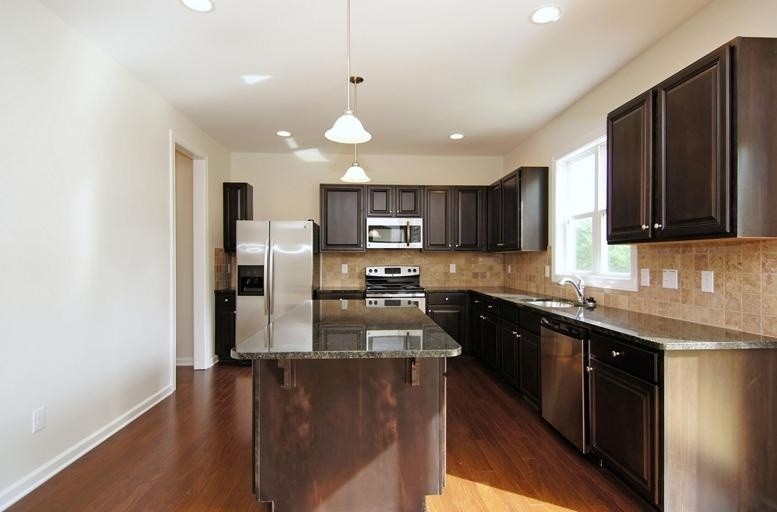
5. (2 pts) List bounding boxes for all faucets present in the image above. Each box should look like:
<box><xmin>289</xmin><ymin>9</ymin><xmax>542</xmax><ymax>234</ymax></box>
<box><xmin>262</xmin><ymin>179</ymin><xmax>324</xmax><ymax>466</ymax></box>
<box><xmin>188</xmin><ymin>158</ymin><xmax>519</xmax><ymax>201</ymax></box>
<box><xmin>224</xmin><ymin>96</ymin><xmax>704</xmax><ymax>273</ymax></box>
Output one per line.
<box><xmin>556</xmin><ymin>274</ymin><xmax>585</xmax><ymax>304</ymax></box>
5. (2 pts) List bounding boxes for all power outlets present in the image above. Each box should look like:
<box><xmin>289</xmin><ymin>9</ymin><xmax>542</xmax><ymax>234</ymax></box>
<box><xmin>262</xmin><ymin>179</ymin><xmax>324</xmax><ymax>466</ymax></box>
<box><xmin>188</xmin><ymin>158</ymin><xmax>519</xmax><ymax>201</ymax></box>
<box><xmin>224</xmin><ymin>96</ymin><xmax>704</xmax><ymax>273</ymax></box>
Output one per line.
<box><xmin>340</xmin><ymin>148</ymin><xmax>376</xmax><ymax>185</ymax></box>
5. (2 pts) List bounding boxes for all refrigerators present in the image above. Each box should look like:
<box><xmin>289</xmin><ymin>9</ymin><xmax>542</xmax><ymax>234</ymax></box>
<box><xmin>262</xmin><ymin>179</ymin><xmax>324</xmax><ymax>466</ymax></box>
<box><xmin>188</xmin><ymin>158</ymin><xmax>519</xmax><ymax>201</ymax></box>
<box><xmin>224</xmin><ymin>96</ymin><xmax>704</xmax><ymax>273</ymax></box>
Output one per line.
<box><xmin>234</xmin><ymin>218</ymin><xmax>319</xmax><ymax>346</ymax></box>
<box><xmin>236</xmin><ymin>301</ymin><xmax>313</xmax><ymax>354</ymax></box>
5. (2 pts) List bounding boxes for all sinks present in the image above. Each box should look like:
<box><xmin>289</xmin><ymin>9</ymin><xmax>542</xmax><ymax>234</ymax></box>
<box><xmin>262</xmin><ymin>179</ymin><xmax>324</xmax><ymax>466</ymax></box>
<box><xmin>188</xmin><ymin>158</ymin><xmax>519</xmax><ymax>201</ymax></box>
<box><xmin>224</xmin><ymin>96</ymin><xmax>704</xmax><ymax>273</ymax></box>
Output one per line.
<box><xmin>527</xmin><ymin>301</ymin><xmax>574</xmax><ymax>308</ymax></box>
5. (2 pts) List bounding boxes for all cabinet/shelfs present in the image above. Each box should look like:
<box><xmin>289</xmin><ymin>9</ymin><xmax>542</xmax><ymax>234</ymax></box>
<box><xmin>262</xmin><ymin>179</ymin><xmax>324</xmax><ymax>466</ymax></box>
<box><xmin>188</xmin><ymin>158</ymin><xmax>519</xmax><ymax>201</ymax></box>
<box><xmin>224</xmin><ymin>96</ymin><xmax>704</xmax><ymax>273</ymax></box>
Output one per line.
<box><xmin>489</xmin><ymin>166</ymin><xmax>547</xmax><ymax>252</ymax></box>
<box><xmin>583</xmin><ymin>314</ymin><xmax>777</xmax><ymax>511</ymax></box>
<box><xmin>215</xmin><ymin>290</ymin><xmax>236</xmax><ymax>359</ymax></box>
<box><xmin>607</xmin><ymin>35</ymin><xmax>777</xmax><ymax>244</ymax></box>
<box><xmin>320</xmin><ymin>184</ymin><xmax>362</xmax><ymax>251</ymax></box>
<box><xmin>499</xmin><ymin>303</ymin><xmax>543</xmax><ymax>402</ymax></box>
<box><xmin>467</xmin><ymin>286</ymin><xmax>499</xmax><ymax>376</ymax></box>
<box><xmin>425</xmin><ymin>184</ymin><xmax>487</xmax><ymax>252</ymax></box>
<box><xmin>425</xmin><ymin>290</ymin><xmax>463</xmax><ymax>350</ymax></box>
<box><xmin>424</xmin><ymin>325</ymin><xmax>458</xmax><ymax>350</ymax></box>
<box><xmin>219</xmin><ymin>182</ymin><xmax>254</xmax><ymax>252</ymax></box>
<box><xmin>319</xmin><ymin>322</ymin><xmax>366</xmax><ymax>352</ymax></box>
<box><xmin>230</xmin><ymin>299</ymin><xmax>468</xmax><ymax>512</ymax></box>
<box><xmin>367</xmin><ymin>186</ymin><xmax>418</xmax><ymax>216</ymax></box>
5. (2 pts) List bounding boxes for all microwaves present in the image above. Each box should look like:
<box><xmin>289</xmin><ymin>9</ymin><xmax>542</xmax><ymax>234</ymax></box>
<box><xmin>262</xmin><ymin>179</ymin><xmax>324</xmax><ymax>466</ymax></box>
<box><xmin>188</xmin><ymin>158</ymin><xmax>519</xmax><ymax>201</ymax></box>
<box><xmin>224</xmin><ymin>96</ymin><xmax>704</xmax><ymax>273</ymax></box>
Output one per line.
<box><xmin>366</xmin><ymin>216</ymin><xmax>423</xmax><ymax>249</ymax></box>
<box><xmin>365</xmin><ymin>328</ymin><xmax>424</xmax><ymax>351</ymax></box>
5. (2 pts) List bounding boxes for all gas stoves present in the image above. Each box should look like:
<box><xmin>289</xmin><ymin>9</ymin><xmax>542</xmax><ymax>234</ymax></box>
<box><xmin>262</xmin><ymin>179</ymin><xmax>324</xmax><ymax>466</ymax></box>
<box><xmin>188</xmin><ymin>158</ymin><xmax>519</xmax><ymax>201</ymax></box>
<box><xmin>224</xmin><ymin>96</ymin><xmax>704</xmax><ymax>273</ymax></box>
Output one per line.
<box><xmin>368</xmin><ymin>299</ymin><xmax>416</xmax><ymax>308</ymax></box>
<box><xmin>365</xmin><ymin>265</ymin><xmax>426</xmax><ymax>298</ymax></box>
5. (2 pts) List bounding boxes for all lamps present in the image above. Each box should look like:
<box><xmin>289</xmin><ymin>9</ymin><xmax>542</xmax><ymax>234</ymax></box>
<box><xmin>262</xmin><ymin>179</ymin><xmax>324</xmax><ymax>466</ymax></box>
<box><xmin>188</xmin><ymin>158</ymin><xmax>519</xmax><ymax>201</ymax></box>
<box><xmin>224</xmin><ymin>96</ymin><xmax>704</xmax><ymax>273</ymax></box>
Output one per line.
<box><xmin>325</xmin><ymin>0</ymin><xmax>369</xmax><ymax>148</ymax></box>
